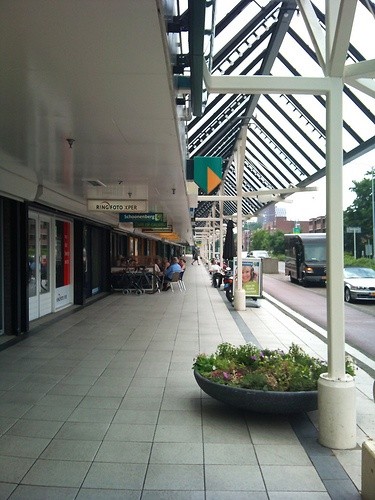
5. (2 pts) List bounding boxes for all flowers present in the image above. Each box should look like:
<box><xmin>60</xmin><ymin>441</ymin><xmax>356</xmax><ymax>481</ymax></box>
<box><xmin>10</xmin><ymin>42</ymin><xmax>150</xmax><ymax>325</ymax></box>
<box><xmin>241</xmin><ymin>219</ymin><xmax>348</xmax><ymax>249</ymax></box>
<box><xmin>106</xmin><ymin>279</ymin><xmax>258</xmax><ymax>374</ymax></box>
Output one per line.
<box><xmin>191</xmin><ymin>342</ymin><xmax>359</xmax><ymax>391</ymax></box>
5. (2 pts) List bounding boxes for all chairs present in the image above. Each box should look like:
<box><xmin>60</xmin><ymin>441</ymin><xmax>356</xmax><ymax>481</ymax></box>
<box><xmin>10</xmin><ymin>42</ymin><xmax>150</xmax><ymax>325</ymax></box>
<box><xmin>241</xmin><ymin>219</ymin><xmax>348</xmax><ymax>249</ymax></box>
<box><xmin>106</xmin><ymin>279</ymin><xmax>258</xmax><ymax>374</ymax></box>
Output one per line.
<box><xmin>165</xmin><ymin>268</ymin><xmax>186</xmax><ymax>294</ymax></box>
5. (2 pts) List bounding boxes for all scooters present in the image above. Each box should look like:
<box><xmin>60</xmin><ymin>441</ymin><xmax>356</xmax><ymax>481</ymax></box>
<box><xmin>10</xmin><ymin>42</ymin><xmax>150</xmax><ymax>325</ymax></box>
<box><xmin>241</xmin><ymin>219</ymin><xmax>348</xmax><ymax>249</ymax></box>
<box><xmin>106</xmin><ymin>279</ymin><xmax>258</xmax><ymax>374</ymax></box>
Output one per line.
<box><xmin>221</xmin><ymin>273</ymin><xmax>258</xmax><ymax>303</ymax></box>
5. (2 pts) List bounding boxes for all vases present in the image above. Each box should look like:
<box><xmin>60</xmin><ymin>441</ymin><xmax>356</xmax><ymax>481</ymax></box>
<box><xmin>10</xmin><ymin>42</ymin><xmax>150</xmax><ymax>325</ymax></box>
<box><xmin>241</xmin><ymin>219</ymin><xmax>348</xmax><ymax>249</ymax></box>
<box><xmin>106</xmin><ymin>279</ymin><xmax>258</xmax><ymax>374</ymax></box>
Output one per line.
<box><xmin>193</xmin><ymin>367</ymin><xmax>318</xmax><ymax>414</ymax></box>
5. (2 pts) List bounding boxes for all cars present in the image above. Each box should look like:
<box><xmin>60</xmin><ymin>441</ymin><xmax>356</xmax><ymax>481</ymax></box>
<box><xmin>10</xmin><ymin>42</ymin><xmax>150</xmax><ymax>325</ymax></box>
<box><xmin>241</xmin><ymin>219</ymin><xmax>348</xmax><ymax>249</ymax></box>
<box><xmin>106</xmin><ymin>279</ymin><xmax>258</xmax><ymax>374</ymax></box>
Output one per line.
<box><xmin>344</xmin><ymin>267</ymin><xmax>375</xmax><ymax>303</ymax></box>
<box><xmin>248</xmin><ymin>250</ymin><xmax>269</xmax><ymax>266</ymax></box>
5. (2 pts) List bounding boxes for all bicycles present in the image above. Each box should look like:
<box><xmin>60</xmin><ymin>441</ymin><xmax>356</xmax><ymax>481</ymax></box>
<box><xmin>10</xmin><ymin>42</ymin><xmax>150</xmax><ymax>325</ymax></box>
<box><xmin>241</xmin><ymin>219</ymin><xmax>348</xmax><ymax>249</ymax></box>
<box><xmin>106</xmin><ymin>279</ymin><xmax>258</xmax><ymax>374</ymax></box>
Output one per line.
<box><xmin>105</xmin><ymin>256</ymin><xmax>162</xmax><ymax>294</ymax></box>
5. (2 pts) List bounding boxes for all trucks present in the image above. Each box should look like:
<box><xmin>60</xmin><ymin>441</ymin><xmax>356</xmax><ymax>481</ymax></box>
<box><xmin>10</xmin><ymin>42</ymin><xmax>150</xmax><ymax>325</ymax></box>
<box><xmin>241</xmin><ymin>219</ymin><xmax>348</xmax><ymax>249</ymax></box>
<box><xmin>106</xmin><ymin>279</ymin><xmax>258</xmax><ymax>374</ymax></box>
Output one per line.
<box><xmin>282</xmin><ymin>233</ymin><xmax>327</xmax><ymax>287</ymax></box>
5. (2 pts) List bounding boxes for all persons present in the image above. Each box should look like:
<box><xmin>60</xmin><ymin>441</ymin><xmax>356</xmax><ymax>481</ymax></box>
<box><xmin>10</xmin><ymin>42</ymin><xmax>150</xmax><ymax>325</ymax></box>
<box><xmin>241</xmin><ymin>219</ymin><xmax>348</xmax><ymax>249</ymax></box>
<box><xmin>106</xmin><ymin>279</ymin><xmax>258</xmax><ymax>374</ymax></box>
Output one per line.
<box><xmin>116</xmin><ymin>248</ymin><xmax>231</xmax><ymax>291</ymax></box>
<box><xmin>236</xmin><ymin>266</ymin><xmax>258</xmax><ymax>287</ymax></box>
